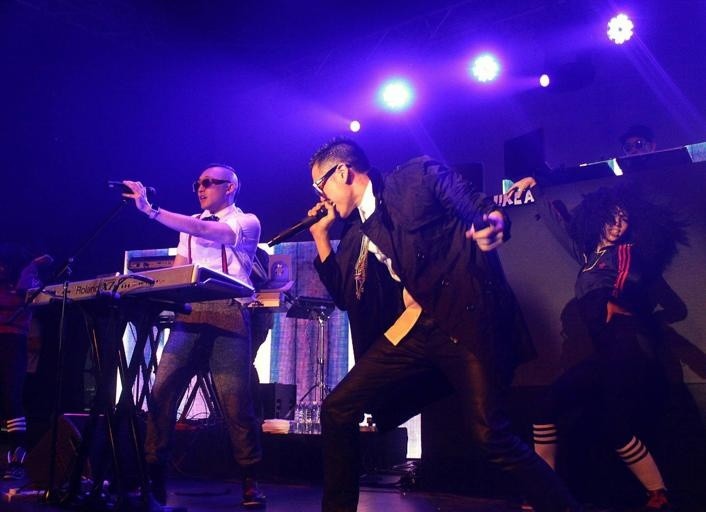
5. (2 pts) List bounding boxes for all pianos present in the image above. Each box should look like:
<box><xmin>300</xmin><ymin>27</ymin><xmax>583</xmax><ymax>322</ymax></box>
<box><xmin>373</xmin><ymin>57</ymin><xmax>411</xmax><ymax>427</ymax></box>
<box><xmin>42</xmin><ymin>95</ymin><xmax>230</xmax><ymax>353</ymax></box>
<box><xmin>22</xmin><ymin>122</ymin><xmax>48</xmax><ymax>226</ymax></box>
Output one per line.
<box><xmin>25</xmin><ymin>263</ymin><xmax>255</xmax><ymax>309</ymax></box>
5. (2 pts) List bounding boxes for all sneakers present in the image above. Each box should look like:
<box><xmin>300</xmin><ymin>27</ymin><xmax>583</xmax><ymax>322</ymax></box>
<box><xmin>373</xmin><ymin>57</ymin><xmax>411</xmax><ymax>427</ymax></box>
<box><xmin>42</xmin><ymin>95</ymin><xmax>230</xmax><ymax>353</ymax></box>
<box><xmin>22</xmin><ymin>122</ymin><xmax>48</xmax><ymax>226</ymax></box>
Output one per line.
<box><xmin>243</xmin><ymin>476</ymin><xmax>266</xmax><ymax>504</ymax></box>
<box><xmin>646</xmin><ymin>487</ymin><xmax>668</xmax><ymax>512</ymax></box>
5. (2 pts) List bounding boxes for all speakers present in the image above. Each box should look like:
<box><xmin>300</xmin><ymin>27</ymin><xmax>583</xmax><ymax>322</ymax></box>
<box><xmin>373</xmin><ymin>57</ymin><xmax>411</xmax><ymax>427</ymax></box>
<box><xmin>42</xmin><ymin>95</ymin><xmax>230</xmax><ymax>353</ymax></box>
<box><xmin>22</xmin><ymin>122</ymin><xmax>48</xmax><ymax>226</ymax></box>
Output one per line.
<box><xmin>258</xmin><ymin>382</ymin><xmax>297</xmax><ymax>422</ymax></box>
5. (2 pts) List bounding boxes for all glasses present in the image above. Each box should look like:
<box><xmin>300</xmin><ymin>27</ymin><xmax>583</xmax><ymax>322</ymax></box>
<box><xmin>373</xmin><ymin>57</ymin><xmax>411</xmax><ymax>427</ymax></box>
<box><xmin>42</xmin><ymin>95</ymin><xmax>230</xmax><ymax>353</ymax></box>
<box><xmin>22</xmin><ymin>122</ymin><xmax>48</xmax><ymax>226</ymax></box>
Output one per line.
<box><xmin>192</xmin><ymin>180</ymin><xmax>231</xmax><ymax>194</ymax></box>
<box><xmin>313</xmin><ymin>163</ymin><xmax>351</xmax><ymax>198</ymax></box>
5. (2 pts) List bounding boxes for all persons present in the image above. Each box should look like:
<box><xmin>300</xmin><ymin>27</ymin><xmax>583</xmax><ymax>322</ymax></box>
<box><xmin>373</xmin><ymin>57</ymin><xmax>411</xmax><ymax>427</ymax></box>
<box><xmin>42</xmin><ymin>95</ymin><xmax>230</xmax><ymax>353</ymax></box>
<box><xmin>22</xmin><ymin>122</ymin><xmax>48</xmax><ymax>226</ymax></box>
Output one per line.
<box><xmin>308</xmin><ymin>134</ymin><xmax>583</xmax><ymax>511</ymax></box>
<box><xmin>506</xmin><ymin>175</ymin><xmax>689</xmax><ymax>511</ymax></box>
<box><xmin>618</xmin><ymin>123</ymin><xmax>658</xmax><ymax>155</ymax></box>
<box><xmin>116</xmin><ymin>162</ymin><xmax>271</xmax><ymax>507</ymax></box>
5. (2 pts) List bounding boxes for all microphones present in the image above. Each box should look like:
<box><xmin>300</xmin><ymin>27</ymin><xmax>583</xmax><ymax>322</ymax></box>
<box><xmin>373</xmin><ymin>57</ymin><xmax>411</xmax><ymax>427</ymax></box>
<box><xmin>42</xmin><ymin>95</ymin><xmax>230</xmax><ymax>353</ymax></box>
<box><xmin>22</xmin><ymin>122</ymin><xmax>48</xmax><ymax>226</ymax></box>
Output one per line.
<box><xmin>268</xmin><ymin>207</ymin><xmax>328</xmax><ymax>246</ymax></box>
<box><xmin>106</xmin><ymin>179</ymin><xmax>156</xmax><ymax>199</ymax></box>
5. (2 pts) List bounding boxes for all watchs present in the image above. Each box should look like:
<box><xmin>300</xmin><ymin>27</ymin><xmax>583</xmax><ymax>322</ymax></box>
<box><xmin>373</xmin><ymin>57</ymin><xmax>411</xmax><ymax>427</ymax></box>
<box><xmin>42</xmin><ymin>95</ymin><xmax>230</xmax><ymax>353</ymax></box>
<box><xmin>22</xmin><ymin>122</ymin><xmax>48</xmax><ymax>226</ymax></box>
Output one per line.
<box><xmin>148</xmin><ymin>203</ymin><xmax>161</xmax><ymax>220</ymax></box>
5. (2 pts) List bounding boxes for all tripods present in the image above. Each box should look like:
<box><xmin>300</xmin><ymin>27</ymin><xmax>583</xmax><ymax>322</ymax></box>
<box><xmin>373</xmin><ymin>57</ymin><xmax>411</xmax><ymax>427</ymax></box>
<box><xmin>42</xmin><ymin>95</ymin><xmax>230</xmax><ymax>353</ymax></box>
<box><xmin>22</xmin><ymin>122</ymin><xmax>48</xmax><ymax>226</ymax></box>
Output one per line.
<box><xmin>284</xmin><ymin>320</ymin><xmax>331</xmax><ymax>421</ymax></box>
